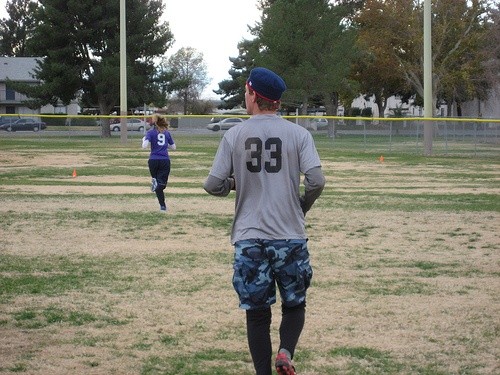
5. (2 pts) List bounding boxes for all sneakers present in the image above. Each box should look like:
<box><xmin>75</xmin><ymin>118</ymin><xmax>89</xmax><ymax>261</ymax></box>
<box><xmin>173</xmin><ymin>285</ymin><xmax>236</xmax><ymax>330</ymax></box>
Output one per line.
<box><xmin>151</xmin><ymin>178</ymin><xmax>158</xmax><ymax>192</ymax></box>
<box><xmin>160</xmin><ymin>205</ymin><xmax>167</xmax><ymax>212</ymax></box>
<box><xmin>275</xmin><ymin>353</ymin><xmax>297</xmax><ymax>375</ymax></box>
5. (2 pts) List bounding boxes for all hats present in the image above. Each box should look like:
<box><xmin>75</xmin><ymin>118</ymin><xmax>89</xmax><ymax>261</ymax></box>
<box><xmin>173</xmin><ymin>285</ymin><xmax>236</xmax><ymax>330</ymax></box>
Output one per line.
<box><xmin>246</xmin><ymin>67</ymin><xmax>287</xmax><ymax>103</ymax></box>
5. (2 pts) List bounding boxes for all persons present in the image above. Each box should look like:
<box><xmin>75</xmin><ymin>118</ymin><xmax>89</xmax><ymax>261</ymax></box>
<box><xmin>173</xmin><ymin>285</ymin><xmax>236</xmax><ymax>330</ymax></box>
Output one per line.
<box><xmin>142</xmin><ymin>115</ymin><xmax>176</xmax><ymax>211</ymax></box>
<box><xmin>203</xmin><ymin>67</ymin><xmax>326</xmax><ymax>375</ymax></box>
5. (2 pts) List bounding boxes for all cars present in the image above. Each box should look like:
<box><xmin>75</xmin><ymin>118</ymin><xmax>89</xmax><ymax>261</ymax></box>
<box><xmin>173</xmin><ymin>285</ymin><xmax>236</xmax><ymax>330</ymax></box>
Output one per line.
<box><xmin>205</xmin><ymin>117</ymin><xmax>245</xmax><ymax>131</ymax></box>
<box><xmin>303</xmin><ymin>118</ymin><xmax>328</xmax><ymax>131</ymax></box>
<box><xmin>135</xmin><ymin>107</ymin><xmax>150</xmax><ymax>116</ymax></box>
<box><xmin>110</xmin><ymin>118</ymin><xmax>150</xmax><ymax>132</ymax></box>
<box><xmin>1</xmin><ymin>119</ymin><xmax>47</xmax><ymax>132</ymax></box>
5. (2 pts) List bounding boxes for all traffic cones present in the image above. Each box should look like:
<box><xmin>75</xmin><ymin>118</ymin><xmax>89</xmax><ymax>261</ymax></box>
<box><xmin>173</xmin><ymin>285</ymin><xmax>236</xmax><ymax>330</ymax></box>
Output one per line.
<box><xmin>380</xmin><ymin>154</ymin><xmax>385</xmax><ymax>162</ymax></box>
<box><xmin>73</xmin><ymin>169</ymin><xmax>77</xmax><ymax>177</ymax></box>
